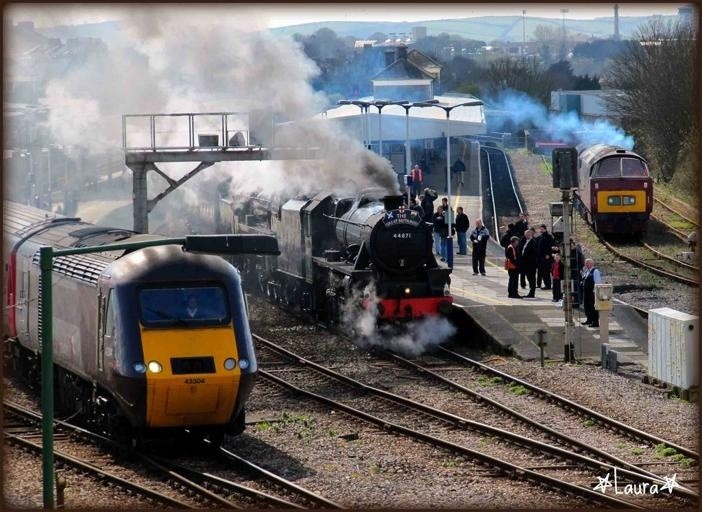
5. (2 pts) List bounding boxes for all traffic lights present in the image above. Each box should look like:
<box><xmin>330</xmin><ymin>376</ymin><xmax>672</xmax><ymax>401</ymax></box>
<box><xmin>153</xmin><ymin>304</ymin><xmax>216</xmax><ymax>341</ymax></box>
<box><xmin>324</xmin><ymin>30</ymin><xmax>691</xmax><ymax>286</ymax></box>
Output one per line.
<box><xmin>593</xmin><ymin>283</ymin><xmax>615</xmax><ymax>310</ymax></box>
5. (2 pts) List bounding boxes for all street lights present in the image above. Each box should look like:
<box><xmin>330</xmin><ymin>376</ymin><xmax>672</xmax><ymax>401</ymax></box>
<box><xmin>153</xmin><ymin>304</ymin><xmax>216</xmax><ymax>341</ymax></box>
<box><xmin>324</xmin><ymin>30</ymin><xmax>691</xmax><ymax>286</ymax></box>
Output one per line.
<box><xmin>559</xmin><ymin>8</ymin><xmax>569</xmax><ymax>42</ymax></box>
<box><xmin>415</xmin><ymin>100</ymin><xmax>485</xmax><ymax>268</ymax></box>
<box><xmin>42</xmin><ymin>147</ymin><xmax>52</xmax><ymax>194</ymax></box>
<box><xmin>521</xmin><ymin>9</ymin><xmax>526</xmax><ymax>54</ymax></box>
<box><xmin>33</xmin><ymin>234</ymin><xmax>280</xmax><ymax>509</ymax></box>
<box><xmin>338</xmin><ymin>99</ymin><xmax>408</xmax><ymax>156</ymax></box>
<box><xmin>376</xmin><ymin>100</ymin><xmax>440</xmax><ymax>210</ymax></box>
<box><xmin>21</xmin><ymin>151</ymin><xmax>35</xmax><ymax>192</ymax></box>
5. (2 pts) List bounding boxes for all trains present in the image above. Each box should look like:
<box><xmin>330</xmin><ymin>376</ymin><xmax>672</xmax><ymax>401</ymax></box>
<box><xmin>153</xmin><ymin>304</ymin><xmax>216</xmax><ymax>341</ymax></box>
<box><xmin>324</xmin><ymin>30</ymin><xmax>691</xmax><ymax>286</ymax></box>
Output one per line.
<box><xmin>576</xmin><ymin>142</ymin><xmax>659</xmax><ymax>247</ymax></box>
<box><xmin>2</xmin><ymin>142</ymin><xmax>256</xmax><ymax>447</ymax></box>
<box><xmin>157</xmin><ymin>163</ymin><xmax>459</xmax><ymax>353</ymax></box>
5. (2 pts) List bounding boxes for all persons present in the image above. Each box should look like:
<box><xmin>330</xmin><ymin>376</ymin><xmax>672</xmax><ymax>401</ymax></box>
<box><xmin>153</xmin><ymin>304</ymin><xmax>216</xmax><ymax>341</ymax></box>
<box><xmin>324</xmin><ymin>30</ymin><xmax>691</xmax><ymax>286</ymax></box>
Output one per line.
<box><xmin>410</xmin><ymin>165</ymin><xmax>424</xmax><ymax>198</ymax></box>
<box><xmin>401</xmin><ymin>185</ymin><xmax>602</xmax><ymax>327</ymax></box>
<box><xmin>452</xmin><ymin>156</ymin><xmax>465</xmax><ymax>187</ymax></box>
<box><xmin>179</xmin><ymin>292</ymin><xmax>207</xmax><ymax>318</ymax></box>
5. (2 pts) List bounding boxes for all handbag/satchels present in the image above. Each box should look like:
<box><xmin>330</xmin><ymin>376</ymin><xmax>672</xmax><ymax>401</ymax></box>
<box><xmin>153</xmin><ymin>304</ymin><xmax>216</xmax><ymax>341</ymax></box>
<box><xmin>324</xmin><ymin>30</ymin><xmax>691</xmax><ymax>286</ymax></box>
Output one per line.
<box><xmin>506</xmin><ymin>259</ymin><xmax>519</xmax><ymax>270</ymax></box>
